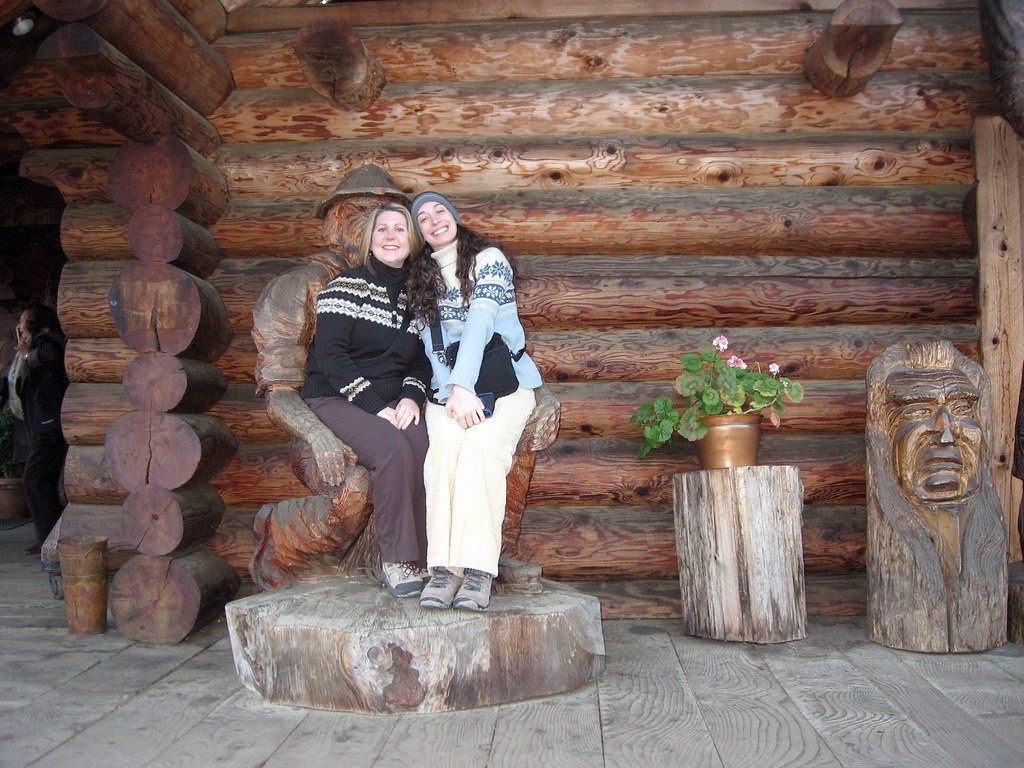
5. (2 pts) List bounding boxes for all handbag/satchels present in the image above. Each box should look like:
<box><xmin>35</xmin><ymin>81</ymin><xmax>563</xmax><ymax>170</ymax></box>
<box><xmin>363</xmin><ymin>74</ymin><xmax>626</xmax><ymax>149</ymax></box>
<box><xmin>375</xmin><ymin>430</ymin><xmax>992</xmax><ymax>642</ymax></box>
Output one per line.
<box><xmin>447</xmin><ymin>332</ymin><xmax>519</xmax><ymax>399</ymax></box>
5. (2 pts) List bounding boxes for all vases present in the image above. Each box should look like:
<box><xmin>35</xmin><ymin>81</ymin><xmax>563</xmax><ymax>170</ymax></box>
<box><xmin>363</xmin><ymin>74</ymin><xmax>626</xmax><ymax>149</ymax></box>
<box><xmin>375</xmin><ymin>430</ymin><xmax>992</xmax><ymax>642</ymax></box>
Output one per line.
<box><xmin>691</xmin><ymin>413</ymin><xmax>762</xmax><ymax>469</ymax></box>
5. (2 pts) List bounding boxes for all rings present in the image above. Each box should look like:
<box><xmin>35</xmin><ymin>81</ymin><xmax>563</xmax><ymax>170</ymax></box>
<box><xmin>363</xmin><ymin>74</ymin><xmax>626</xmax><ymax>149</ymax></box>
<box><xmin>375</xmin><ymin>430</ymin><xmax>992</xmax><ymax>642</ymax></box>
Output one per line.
<box><xmin>409</xmin><ymin>413</ymin><xmax>414</xmax><ymax>417</ymax></box>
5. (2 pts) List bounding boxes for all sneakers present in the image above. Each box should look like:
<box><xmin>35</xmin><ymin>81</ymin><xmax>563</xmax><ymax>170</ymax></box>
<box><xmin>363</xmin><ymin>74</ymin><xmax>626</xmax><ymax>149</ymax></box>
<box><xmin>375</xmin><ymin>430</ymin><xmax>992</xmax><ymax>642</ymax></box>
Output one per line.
<box><xmin>453</xmin><ymin>568</ymin><xmax>493</xmax><ymax>612</ymax></box>
<box><xmin>419</xmin><ymin>566</ymin><xmax>463</xmax><ymax>609</ymax></box>
<box><xmin>381</xmin><ymin>560</ymin><xmax>430</xmax><ymax>598</ymax></box>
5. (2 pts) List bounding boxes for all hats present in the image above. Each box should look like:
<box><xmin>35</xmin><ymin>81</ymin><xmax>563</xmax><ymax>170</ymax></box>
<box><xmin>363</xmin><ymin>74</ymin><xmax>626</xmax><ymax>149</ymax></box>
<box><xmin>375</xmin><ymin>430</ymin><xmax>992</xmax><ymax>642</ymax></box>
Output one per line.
<box><xmin>410</xmin><ymin>191</ymin><xmax>460</xmax><ymax>244</ymax></box>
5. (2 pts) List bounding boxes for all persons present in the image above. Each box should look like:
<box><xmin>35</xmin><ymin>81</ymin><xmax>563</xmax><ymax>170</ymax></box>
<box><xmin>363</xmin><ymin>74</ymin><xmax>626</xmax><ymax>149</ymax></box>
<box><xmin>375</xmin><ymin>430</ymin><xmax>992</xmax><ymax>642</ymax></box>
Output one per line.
<box><xmin>404</xmin><ymin>191</ymin><xmax>543</xmax><ymax>614</ymax></box>
<box><xmin>860</xmin><ymin>331</ymin><xmax>1011</xmax><ymax>654</ymax></box>
<box><xmin>247</xmin><ymin>163</ymin><xmax>561</xmax><ymax>596</ymax></box>
<box><xmin>303</xmin><ymin>203</ymin><xmax>433</xmax><ymax>598</ymax></box>
<box><xmin>0</xmin><ymin>301</ymin><xmax>69</xmax><ymax>555</ymax></box>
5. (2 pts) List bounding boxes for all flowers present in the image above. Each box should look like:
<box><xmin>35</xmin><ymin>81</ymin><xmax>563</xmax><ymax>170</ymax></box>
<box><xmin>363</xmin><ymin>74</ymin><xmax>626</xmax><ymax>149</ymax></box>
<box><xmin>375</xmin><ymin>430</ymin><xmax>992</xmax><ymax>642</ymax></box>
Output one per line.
<box><xmin>629</xmin><ymin>334</ymin><xmax>804</xmax><ymax>459</ymax></box>
